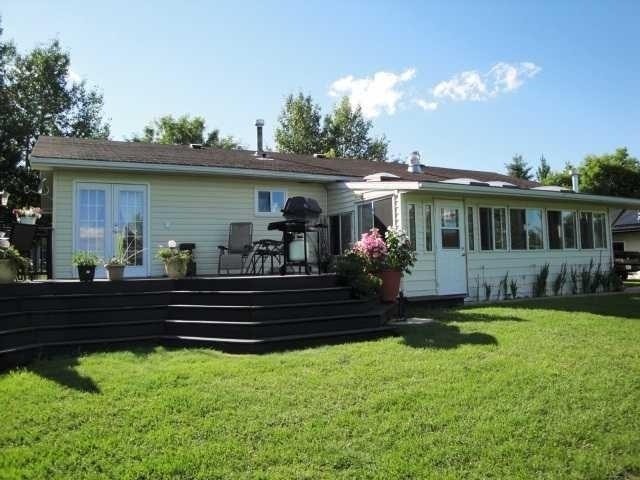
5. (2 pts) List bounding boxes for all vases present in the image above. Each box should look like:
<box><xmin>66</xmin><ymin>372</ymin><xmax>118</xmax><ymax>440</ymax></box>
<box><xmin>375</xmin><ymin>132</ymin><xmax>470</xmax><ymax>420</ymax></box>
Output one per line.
<box><xmin>17</xmin><ymin>216</ymin><xmax>37</xmax><ymax>225</ymax></box>
<box><xmin>376</xmin><ymin>269</ymin><xmax>401</xmax><ymax>303</ymax></box>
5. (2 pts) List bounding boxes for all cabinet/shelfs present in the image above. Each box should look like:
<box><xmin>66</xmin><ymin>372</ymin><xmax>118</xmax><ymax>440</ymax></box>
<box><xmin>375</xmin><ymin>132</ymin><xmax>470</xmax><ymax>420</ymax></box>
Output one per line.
<box><xmin>280</xmin><ymin>224</ymin><xmax>324</xmax><ymax>275</ymax></box>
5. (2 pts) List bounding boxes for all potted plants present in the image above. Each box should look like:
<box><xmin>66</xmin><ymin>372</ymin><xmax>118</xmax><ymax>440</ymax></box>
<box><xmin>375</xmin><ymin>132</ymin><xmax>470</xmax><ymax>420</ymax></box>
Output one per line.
<box><xmin>158</xmin><ymin>246</ymin><xmax>192</xmax><ymax>279</ymax></box>
<box><xmin>0</xmin><ymin>245</ymin><xmax>33</xmax><ymax>282</ymax></box>
<box><xmin>103</xmin><ymin>229</ymin><xmax>146</xmax><ymax>280</ymax></box>
<box><xmin>71</xmin><ymin>250</ymin><xmax>99</xmax><ymax>282</ymax></box>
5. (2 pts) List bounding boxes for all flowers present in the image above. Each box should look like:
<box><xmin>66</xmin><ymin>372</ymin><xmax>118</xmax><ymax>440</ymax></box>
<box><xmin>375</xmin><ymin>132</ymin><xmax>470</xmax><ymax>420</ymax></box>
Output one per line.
<box><xmin>12</xmin><ymin>205</ymin><xmax>44</xmax><ymax>225</ymax></box>
<box><xmin>352</xmin><ymin>226</ymin><xmax>416</xmax><ymax>273</ymax></box>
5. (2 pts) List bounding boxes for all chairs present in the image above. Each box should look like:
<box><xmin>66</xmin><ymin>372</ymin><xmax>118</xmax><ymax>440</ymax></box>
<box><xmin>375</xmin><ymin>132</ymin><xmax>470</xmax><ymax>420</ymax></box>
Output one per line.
<box><xmin>254</xmin><ymin>232</ymin><xmax>296</xmax><ymax>273</ymax></box>
<box><xmin>217</xmin><ymin>221</ymin><xmax>257</xmax><ymax>276</ymax></box>
